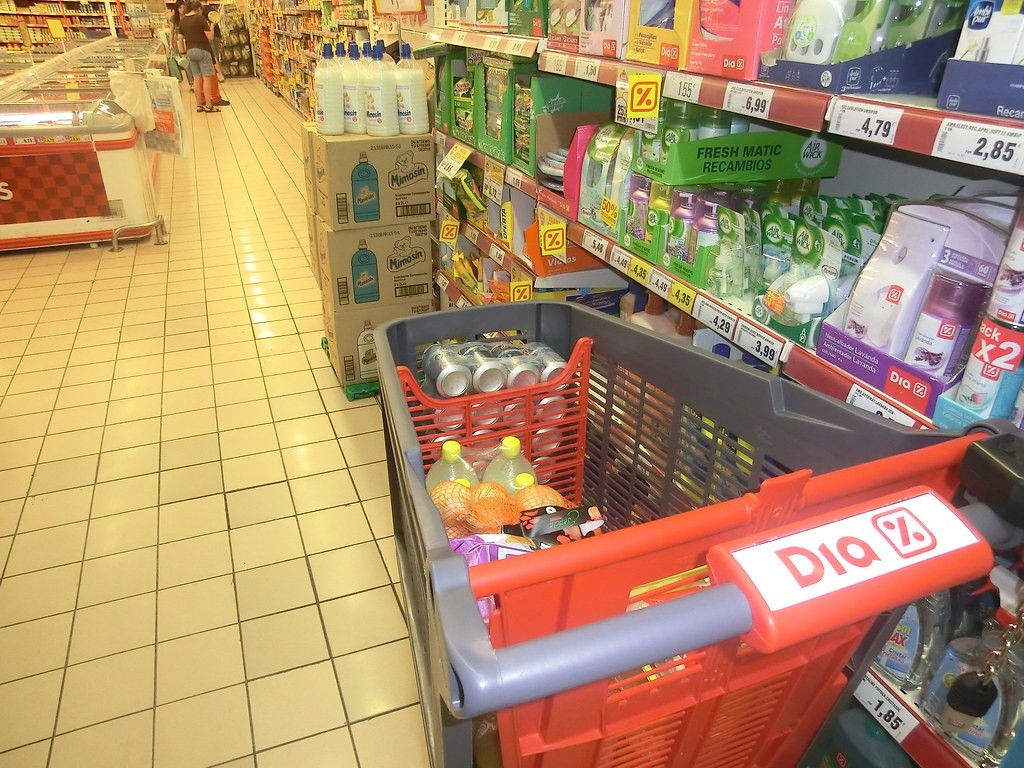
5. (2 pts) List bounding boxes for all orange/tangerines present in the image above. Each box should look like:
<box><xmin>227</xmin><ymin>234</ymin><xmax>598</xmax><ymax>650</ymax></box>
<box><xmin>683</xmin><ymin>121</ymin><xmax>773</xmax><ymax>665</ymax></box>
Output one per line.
<box><xmin>433</xmin><ymin>481</ymin><xmax>564</xmax><ymax>539</ymax></box>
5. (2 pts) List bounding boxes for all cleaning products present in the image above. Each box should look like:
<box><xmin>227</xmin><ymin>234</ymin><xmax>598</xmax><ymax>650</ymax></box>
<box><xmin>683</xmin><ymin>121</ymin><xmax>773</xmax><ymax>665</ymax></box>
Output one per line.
<box><xmin>869</xmin><ymin>546</ymin><xmax>1023</xmax><ymax>768</ymax></box>
<box><xmin>815</xmin><ymin>709</ymin><xmax>912</xmax><ymax>768</ymax></box>
<box><xmin>314</xmin><ymin>39</ymin><xmax>430</xmax><ymax>136</ymax></box>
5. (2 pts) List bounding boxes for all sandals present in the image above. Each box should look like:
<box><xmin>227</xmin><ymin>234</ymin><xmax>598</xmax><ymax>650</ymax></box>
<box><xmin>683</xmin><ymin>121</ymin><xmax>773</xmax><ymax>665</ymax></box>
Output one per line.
<box><xmin>197</xmin><ymin>105</ymin><xmax>205</xmax><ymax>112</ymax></box>
<box><xmin>205</xmin><ymin>106</ymin><xmax>221</xmax><ymax>112</ymax></box>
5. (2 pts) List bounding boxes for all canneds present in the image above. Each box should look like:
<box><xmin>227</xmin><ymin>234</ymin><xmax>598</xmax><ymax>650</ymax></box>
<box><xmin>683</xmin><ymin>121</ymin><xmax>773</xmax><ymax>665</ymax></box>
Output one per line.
<box><xmin>421</xmin><ymin>339</ymin><xmax>568</xmax><ymax>484</ymax></box>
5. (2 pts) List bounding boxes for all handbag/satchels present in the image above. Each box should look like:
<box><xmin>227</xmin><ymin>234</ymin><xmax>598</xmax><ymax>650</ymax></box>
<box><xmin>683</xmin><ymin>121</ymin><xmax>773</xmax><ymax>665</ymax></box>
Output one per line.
<box><xmin>167</xmin><ymin>49</ymin><xmax>183</xmax><ymax>83</ymax></box>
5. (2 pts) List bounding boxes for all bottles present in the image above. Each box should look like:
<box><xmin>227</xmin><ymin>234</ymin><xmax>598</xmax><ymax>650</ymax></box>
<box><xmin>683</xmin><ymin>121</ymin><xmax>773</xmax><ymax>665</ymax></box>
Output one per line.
<box><xmin>351</xmin><ymin>239</ymin><xmax>380</xmax><ymax>304</ymax></box>
<box><xmin>425</xmin><ymin>440</ymin><xmax>538</xmax><ymax>495</ymax></box>
<box><xmin>350</xmin><ymin>152</ymin><xmax>380</xmax><ymax>223</ymax></box>
<box><xmin>316</xmin><ymin>40</ymin><xmax>430</xmax><ymax>137</ymax></box>
<box><xmin>357</xmin><ymin>320</ymin><xmax>378</xmax><ymax>379</ymax></box>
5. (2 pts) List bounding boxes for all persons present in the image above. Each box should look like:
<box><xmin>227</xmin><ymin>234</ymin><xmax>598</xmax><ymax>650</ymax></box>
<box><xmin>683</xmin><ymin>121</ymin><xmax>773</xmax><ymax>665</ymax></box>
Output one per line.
<box><xmin>169</xmin><ymin>0</ymin><xmax>230</xmax><ymax>113</ymax></box>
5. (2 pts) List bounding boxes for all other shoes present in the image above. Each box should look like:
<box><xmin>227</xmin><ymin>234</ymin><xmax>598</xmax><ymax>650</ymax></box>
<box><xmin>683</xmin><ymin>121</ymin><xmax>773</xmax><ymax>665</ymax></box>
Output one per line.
<box><xmin>216</xmin><ymin>73</ymin><xmax>225</xmax><ymax>83</ymax></box>
<box><xmin>190</xmin><ymin>86</ymin><xmax>194</xmax><ymax>91</ymax></box>
<box><xmin>210</xmin><ymin>97</ymin><xmax>230</xmax><ymax>106</ymax></box>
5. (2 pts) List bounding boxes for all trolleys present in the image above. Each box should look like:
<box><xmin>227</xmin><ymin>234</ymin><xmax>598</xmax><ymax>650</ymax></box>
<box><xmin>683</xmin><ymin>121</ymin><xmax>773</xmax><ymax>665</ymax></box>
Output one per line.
<box><xmin>374</xmin><ymin>301</ymin><xmax>1024</xmax><ymax>768</ymax></box>
